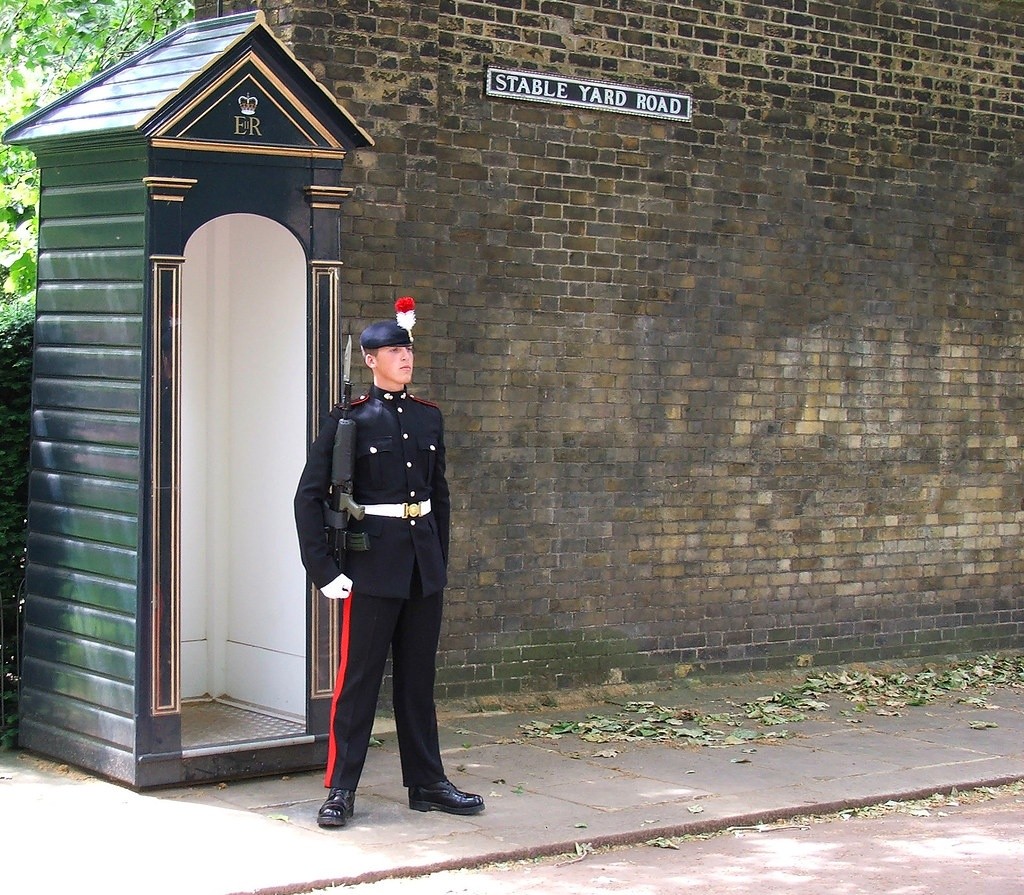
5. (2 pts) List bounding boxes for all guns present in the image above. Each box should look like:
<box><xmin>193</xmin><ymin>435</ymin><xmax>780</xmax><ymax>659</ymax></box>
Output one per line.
<box><xmin>326</xmin><ymin>336</ymin><xmax>356</xmax><ymax>586</ymax></box>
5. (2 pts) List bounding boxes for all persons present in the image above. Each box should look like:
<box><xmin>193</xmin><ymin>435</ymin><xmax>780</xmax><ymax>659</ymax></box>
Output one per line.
<box><xmin>293</xmin><ymin>321</ymin><xmax>486</xmax><ymax>826</ymax></box>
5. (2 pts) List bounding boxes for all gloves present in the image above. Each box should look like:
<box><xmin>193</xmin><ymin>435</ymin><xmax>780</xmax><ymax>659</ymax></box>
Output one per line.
<box><xmin>320</xmin><ymin>573</ymin><xmax>353</xmax><ymax>599</ymax></box>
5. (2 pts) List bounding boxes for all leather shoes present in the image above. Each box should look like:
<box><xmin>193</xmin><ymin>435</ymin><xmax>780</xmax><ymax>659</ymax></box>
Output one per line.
<box><xmin>409</xmin><ymin>780</ymin><xmax>485</xmax><ymax>815</ymax></box>
<box><xmin>317</xmin><ymin>788</ymin><xmax>355</xmax><ymax>825</ymax></box>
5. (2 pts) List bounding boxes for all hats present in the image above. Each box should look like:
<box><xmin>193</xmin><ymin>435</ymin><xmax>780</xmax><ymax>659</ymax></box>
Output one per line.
<box><xmin>360</xmin><ymin>320</ymin><xmax>414</xmax><ymax>349</ymax></box>
